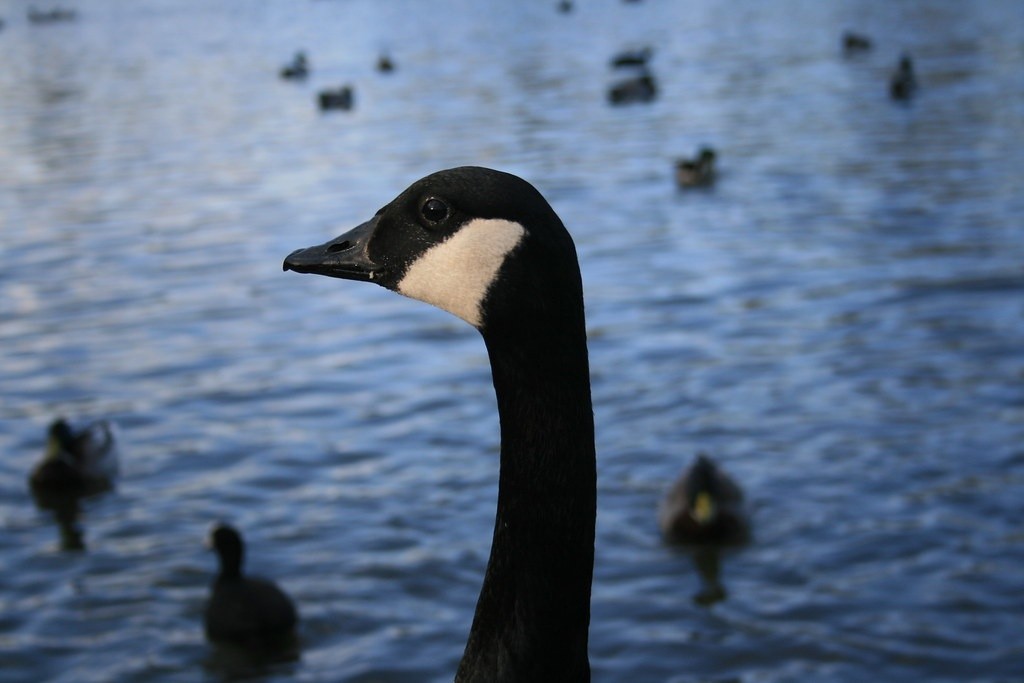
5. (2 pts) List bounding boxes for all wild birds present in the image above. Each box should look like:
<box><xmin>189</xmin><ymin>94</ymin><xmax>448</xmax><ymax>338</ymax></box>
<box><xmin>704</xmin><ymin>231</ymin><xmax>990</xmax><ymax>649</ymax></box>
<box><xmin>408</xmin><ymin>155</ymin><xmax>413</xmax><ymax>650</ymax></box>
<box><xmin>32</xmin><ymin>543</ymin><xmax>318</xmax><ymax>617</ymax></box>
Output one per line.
<box><xmin>45</xmin><ymin>416</ymin><xmax>120</xmax><ymax>484</ymax></box>
<box><xmin>282</xmin><ymin>165</ymin><xmax>597</xmax><ymax>683</ymax></box>
<box><xmin>677</xmin><ymin>147</ymin><xmax>716</xmax><ymax>188</ymax></box>
<box><xmin>660</xmin><ymin>455</ymin><xmax>750</xmax><ymax>547</ymax></box>
<box><xmin>888</xmin><ymin>56</ymin><xmax>915</xmax><ymax>102</ymax></box>
<box><xmin>27</xmin><ymin>456</ymin><xmax>87</xmax><ymax>550</ymax></box>
<box><xmin>202</xmin><ymin>522</ymin><xmax>297</xmax><ymax>651</ymax></box>
